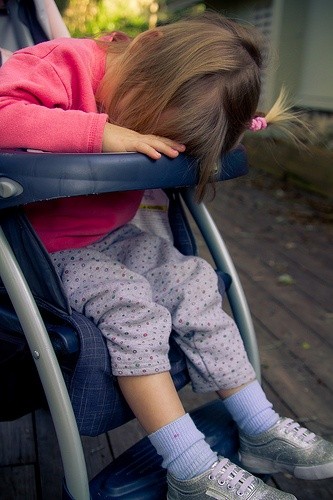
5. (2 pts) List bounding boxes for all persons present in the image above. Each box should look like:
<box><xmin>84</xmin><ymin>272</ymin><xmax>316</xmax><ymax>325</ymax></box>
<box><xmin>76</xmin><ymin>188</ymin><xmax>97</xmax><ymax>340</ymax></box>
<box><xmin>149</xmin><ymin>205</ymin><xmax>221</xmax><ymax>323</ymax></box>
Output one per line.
<box><xmin>0</xmin><ymin>13</ymin><xmax>333</xmax><ymax>500</ymax></box>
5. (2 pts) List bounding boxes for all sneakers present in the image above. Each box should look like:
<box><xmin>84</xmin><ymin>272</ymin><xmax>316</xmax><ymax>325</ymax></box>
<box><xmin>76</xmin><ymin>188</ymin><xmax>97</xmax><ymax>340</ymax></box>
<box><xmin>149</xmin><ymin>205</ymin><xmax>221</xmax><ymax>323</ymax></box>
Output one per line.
<box><xmin>167</xmin><ymin>455</ymin><xmax>297</xmax><ymax>500</ymax></box>
<box><xmin>238</xmin><ymin>417</ymin><xmax>333</xmax><ymax>480</ymax></box>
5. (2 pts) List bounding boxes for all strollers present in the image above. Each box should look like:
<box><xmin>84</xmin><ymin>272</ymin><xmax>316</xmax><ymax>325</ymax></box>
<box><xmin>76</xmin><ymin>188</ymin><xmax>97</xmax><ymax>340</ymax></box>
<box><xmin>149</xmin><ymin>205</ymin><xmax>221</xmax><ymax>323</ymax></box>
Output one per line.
<box><xmin>0</xmin><ymin>1</ymin><xmax>263</xmax><ymax>499</ymax></box>
